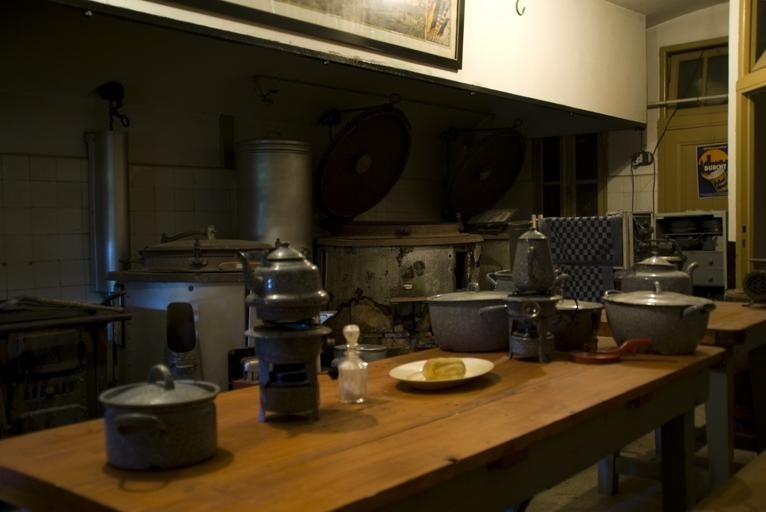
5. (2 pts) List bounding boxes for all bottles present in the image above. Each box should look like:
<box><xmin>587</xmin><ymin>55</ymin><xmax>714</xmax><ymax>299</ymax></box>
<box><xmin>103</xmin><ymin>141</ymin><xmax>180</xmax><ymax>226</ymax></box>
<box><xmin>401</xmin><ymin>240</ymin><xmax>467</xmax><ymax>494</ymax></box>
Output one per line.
<box><xmin>332</xmin><ymin>321</ymin><xmax>370</xmax><ymax>406</ymax></box>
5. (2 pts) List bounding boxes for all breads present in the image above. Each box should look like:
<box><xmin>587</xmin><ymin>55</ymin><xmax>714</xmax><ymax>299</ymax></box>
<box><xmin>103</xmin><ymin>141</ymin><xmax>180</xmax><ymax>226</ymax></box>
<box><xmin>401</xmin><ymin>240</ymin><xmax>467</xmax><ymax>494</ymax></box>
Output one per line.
<box><xmin>422</xmin><ymin>356</ymin><xmax>466</xmax><ymax>382</ymax></box>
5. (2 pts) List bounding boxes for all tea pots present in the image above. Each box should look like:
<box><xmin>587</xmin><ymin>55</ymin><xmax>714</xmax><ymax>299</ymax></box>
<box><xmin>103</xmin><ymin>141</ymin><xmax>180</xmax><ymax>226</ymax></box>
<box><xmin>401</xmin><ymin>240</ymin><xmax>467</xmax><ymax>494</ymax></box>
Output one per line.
<box><xmin>616</xmin><ymin>250</ymin><xmax>702</xmax><ymax>293</ymax></box>
<box><xmin>232</xmin><ymin>238</ymin><xmax>330</xmax><ymax>324</ymax></box>
<box><xmin>510</xmin><ymin>224</ymin><xmax>555</xmax><ymax>291</ymax></box>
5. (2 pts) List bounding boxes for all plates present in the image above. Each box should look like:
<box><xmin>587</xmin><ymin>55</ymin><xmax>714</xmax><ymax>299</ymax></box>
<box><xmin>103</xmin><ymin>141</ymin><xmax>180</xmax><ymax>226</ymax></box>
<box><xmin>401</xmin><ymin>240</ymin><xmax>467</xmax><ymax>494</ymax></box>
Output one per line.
<box><xmin>385</xmin><ymin>355</ymin><xmax>498</xmax><ymax>391</ymax></box>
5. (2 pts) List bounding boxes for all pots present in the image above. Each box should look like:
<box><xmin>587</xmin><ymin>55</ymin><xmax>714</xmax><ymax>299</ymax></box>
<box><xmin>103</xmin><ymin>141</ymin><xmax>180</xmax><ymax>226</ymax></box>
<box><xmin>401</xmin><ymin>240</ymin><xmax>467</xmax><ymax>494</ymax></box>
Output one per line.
<box><xmin>601</xmin><ymin>290</ymin><xmax>717</xmax><ymax>360</ymax></box>
<box><xmin>428</xmin><ymin>282</ymin><xmax>505</xmax><ymax>354</ymax></box>
<box><xmin>551</xmin><ymin>296</ymin><xmax>604</xmax><ymax>356</ymax></box>
<box><xmin>93</xmin><ymin>360</ymin><xmax>226</xmax><ymax>472</ymax></box>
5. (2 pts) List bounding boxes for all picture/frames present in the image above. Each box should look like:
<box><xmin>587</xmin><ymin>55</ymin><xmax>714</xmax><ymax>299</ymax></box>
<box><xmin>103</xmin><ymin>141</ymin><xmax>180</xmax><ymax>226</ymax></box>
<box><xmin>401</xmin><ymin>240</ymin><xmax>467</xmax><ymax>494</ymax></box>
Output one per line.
<box><xmin>167</xmin><ymin>1</ymin><xmax>464</xmax><ymax>74</ymax></box>
